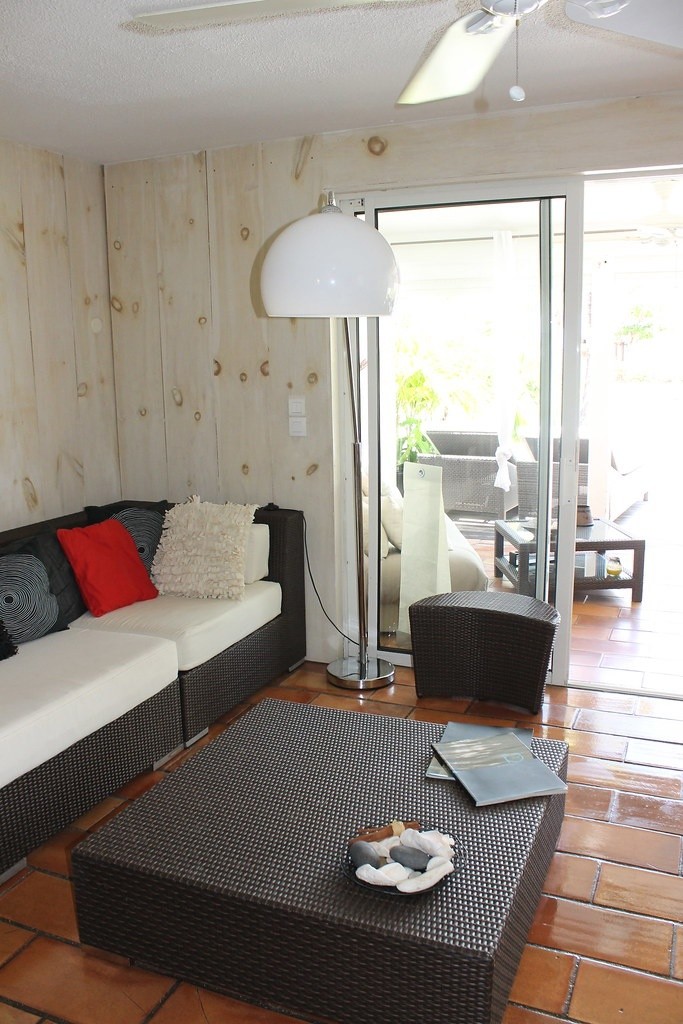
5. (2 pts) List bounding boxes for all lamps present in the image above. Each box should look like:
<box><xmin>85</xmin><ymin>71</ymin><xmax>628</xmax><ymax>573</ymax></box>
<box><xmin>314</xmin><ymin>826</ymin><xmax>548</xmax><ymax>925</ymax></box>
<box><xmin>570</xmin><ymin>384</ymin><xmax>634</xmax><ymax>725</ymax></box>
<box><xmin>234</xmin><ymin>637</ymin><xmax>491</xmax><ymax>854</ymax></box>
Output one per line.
<box><xmin>259</xmin><ymin>189</ymin><xmax>401</xmax><ymax>691</ymax></box>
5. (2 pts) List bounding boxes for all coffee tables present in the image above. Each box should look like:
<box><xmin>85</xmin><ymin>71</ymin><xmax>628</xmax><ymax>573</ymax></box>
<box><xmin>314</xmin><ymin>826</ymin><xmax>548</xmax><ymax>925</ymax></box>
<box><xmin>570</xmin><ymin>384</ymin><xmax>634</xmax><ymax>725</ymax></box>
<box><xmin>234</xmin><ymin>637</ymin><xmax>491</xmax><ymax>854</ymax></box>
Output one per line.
<box><xmin>70</xmin><ymin>697</ymin><xmax>570</xmax><ymax>1024</ymax></box>
<box><xmin>494</xmin><ymin>518</ymin><xmax>645</xmax><ymax>603</ymax></box>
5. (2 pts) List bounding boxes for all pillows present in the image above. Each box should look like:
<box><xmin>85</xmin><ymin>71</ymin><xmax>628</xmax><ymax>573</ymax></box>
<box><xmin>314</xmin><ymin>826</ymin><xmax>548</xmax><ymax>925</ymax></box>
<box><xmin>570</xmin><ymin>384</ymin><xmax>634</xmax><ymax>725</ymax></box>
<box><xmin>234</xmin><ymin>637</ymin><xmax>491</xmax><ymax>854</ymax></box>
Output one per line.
<box><xmin>147</xmin><ymin>493</ymin><xmax>260</xmax><ymax>602</ymax></box>
<box><xmin>54</xmin><ymin>516</ymin><xmax>159</xmax><ymax>620</ymax></box>
<box><xmin>0</xmin><ymin>534</ymin><xmax>71</xmax><ymax>644</ymax></box>
<box><xmin>381</xmin><ymin>476</ymin><xmax>404</xmax><ymax>550</ymax></box>
<box><xmin>84</xmin><ymin>498</ymin><xmax>168</xmax><ymax>577</ymax></box>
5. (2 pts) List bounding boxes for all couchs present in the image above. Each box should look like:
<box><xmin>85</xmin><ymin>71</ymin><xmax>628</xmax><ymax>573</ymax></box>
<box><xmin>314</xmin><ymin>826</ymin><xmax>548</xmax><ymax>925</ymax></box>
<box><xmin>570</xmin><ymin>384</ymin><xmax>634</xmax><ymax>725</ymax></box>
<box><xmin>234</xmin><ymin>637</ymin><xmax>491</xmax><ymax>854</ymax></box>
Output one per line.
<box><xmin>380</xmin><ymin>512</ymin><xmax>490</xmax><ymax>636</ymax></box>
<box><xmin>0</xmin><ymin>501</ymin><xmax>308</xmax><ymax>886</ymax></box>
<box><xmin>417</xmin><ymin>430</ymin><xmax>518</xmax><ymax>520</ymax></box>
<box><xmin>517</xmin><ymin>437</ymin><xmax>652</xmax><ymax>520</ymax></box>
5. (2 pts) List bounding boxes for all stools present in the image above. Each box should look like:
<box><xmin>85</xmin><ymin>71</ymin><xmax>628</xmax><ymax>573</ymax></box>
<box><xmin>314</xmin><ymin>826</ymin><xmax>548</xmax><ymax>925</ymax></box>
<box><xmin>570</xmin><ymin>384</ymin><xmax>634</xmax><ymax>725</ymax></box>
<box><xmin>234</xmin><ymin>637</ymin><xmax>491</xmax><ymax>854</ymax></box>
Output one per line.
<box><xmin>410</xmin><ymin>591</ymin><xmax>562</xmax><ymax>715</ymax></box>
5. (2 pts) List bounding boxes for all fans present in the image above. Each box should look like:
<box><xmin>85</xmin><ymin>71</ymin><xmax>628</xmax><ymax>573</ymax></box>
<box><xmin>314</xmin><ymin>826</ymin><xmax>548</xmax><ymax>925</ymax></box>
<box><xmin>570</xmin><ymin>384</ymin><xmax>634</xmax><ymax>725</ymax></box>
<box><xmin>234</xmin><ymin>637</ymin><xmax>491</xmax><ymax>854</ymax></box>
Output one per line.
<box><xmin>133</xmin><ymin>0</ymin><xmax>683</xmax><ymax>107</ymax></box>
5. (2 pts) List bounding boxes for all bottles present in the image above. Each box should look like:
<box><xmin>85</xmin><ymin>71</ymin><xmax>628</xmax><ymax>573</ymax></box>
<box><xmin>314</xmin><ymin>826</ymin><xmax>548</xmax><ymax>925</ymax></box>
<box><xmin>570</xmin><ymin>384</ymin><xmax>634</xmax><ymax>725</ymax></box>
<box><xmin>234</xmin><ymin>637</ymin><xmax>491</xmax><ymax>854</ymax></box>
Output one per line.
<box><xmin>606</xmin><ymin>557</ymin><xmax>622</xmax><ymax>576</ymax></box>
